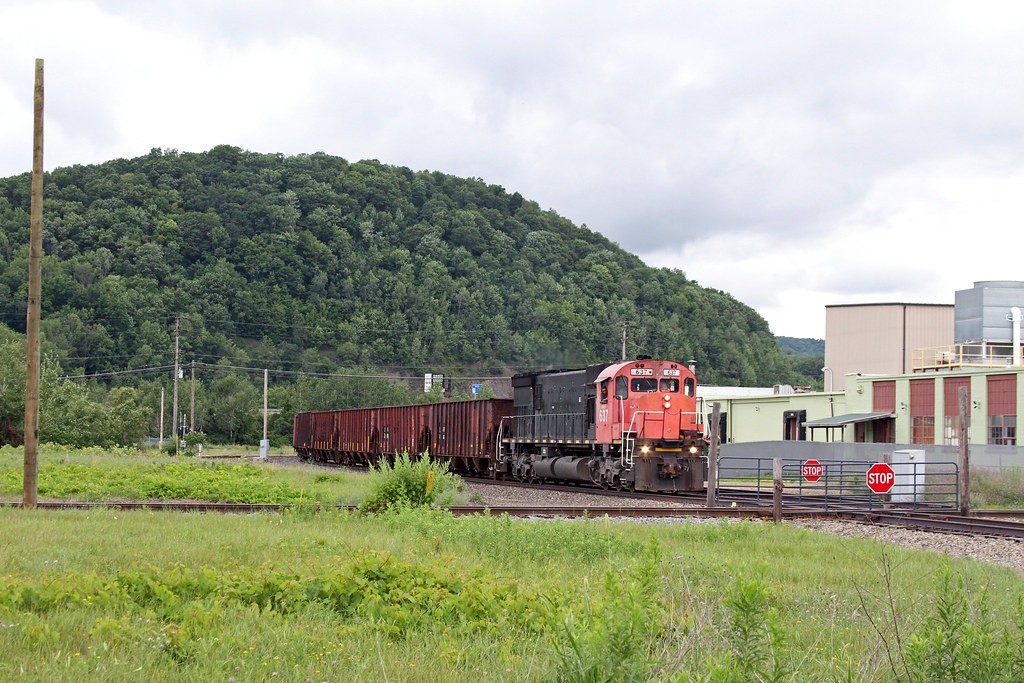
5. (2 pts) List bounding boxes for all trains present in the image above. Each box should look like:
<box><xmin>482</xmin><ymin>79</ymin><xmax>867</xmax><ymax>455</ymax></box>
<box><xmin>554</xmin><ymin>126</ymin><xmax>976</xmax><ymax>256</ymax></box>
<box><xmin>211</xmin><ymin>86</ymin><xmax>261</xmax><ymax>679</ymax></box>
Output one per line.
<box><xmin>292</xmin><ymin>354</ymin><xmax>711</xmax><ymax>496</ymax></box>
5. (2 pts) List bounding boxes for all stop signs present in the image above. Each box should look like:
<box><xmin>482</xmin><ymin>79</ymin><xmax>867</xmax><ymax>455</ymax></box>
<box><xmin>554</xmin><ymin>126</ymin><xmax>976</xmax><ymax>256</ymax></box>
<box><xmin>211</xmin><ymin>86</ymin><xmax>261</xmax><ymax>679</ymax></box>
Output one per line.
<box><xmin>801</xmin><ymin>459</ymin><xmax>824</xmax><ymax>483</ymax></box>
<box><xmin>865</xmin><ymin>463</ymin><xmax>896</xmax><ymax>495</ymax></box>
